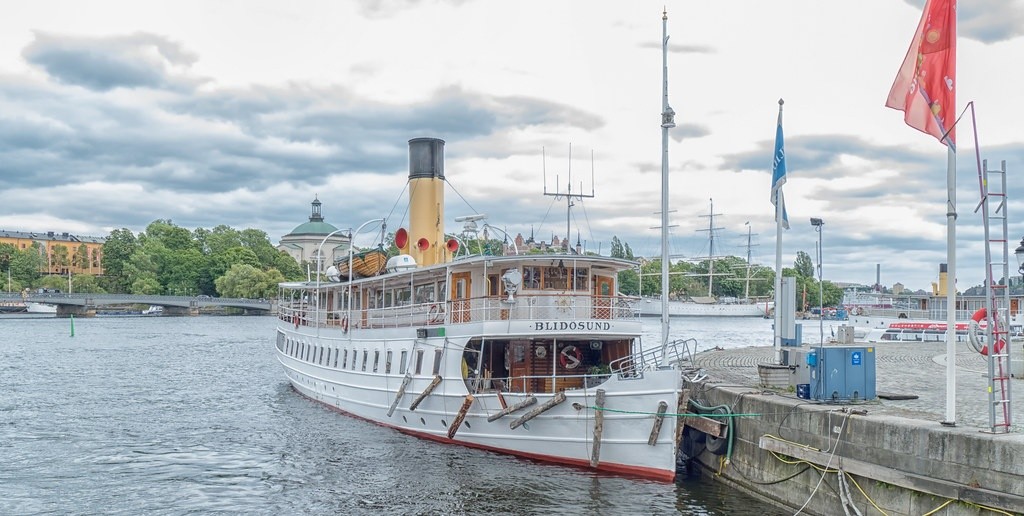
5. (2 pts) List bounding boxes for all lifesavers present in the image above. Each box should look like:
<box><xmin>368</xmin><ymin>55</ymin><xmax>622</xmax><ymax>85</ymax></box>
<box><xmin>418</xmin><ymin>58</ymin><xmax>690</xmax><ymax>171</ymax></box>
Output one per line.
<box><xmin>340</xmin><ymin>315</ymin><xmax>348</xmax><ymax>334</ymax></box>
<box><xmin>968</xmin><ymin>307</ymin><xmax>1008</xmax><ymax>356</ymax></box>
<box><xmin>427</xmin><ymin>304</ymin><xmax>441</xmax><ymax>322</ymax></box>
<box><xmin>294</xmin><ymin>314</ymin><xmax>299</xmax><ymax>328</ymax></box>
<box><xmin>560</xmin><ymin>346</ymin><xmax>581</xmax><ymax>369</ymax></box>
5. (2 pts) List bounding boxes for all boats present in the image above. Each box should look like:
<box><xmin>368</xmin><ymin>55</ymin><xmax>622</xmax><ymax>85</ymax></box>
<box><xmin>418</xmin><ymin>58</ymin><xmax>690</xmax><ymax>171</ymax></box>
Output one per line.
<box><xmin>0</xmin><ymin>297</ymin><xmax>58</xmax><ymax>319</ymax></box>
<box><xmin>331</xmin><ymin>217</ymin><xmax>390</xmax><ymax>280</ymax></box>
<box><xmin>272</xmin><ymin>3</ymin><xmax>707</xmax><ymax>487</ymax></box>
<box><xmin>142</xmin><ymin>304</ymin><xmax>163</xmax><ymax>317</ymax></box>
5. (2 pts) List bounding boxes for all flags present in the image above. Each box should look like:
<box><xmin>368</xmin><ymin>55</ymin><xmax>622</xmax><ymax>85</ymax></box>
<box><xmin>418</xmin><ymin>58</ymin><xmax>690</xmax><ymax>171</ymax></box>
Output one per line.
<box><xmin>769</xmin><ymin>108</ymin><xmax>789</xmax><ymax>230</ymax></box>
<box><xmin>884</xmin><ymin>0</ymin><xmax>957</xmax><ymax>153</ymax></box>
